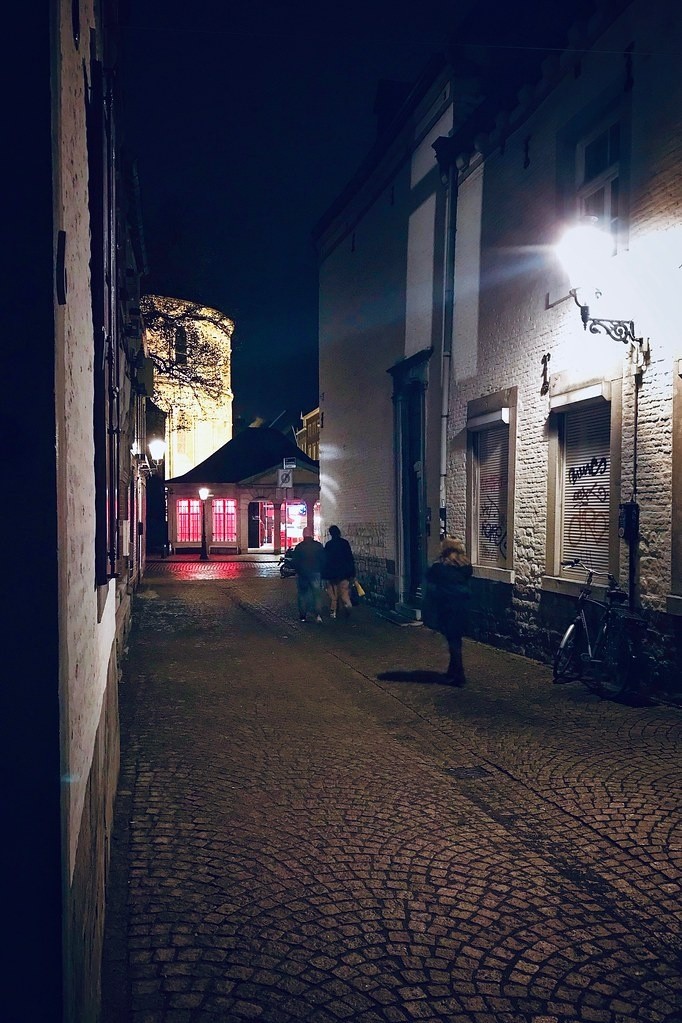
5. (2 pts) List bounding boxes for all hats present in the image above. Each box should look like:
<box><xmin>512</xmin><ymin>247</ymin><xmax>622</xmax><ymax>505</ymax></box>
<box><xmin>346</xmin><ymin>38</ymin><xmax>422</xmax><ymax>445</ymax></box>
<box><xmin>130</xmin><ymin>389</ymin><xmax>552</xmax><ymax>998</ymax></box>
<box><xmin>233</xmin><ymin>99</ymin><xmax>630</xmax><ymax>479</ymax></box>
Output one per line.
<box><xmin>303</xmin><ymin>527</ymin><xmax>313</xmax><ymax>537</ymax></box>
<box><xmin>441</xmin><ymin>538</ymin><xmax>464</xmax><ymax>557</ymax></box>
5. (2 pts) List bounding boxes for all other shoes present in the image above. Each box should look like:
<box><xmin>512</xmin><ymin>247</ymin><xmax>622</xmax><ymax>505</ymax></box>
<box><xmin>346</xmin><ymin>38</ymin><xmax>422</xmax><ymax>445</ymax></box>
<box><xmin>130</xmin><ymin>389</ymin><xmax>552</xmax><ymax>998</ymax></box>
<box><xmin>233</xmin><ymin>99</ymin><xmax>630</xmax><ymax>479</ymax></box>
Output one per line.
<box><xmin>447</xmin><ymin>670</ymin><xmax>454</xmax><ymax>679</ymax></box>
<box><xmin>344</xmin><ymin>600</ymin><xmax>352</xmax><ymax>607</ymax></box>
<box><xmin>330</xmin><ymin>612</ymin><xmax>336</xmax><ymax>618</ymax></box>
<box><xmin>298</xmin><ymin>615</ymin><xmax>307</xmax><ymax>621</ymax></box>
<box><xmin>316</xmin><ymin>616</ymin><xmax>322</xmax><ymax>623</ymax></box>
<box><xmin>451</xmin><ymin>679</ymin><xmax>464</xmax><ymax>687</ymax></box>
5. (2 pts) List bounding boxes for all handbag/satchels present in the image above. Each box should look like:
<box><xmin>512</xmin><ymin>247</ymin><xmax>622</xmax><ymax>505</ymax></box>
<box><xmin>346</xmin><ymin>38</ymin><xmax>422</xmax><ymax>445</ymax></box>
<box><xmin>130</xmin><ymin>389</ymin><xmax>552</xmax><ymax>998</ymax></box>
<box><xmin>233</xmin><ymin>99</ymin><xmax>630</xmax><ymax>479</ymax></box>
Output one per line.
<box><xmin>350</xmin><ymin>587</ymin><xmax>361</xmax><ymax>606</ymax></box>
<box><xmin>354</xmin><ymin>579</ymin><xmax>366</xmax><ymax>597</ymax></box>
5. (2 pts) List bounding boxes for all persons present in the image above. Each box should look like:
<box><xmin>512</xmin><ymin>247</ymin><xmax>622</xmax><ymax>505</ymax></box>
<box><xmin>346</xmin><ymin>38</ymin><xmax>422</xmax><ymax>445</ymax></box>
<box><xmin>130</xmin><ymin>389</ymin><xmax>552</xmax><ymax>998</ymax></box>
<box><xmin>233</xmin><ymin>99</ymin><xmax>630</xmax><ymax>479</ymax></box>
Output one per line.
<box><xmin>325</xmin><ymin>525</ymin><xmax>357</xmax><ymax>620</ymax></box>
<box><xmin>425</xmin><ymin>536</ymin><xmax>474</xmax><ymax>687</ymax></box>
<box><xmin>294</xmin><ymin>527</ymin><xmax>325</xmax><ymax>623</ymax></box>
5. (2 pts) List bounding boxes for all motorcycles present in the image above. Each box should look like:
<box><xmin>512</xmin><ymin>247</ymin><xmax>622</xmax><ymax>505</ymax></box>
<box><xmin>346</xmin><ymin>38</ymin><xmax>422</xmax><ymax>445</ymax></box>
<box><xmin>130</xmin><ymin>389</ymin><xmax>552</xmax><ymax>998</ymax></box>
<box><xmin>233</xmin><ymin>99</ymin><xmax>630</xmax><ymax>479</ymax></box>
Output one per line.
<box><xmin>277</xmin><ymin>546</ymin><xmax>296</xmax><ymax>579</ymax></box>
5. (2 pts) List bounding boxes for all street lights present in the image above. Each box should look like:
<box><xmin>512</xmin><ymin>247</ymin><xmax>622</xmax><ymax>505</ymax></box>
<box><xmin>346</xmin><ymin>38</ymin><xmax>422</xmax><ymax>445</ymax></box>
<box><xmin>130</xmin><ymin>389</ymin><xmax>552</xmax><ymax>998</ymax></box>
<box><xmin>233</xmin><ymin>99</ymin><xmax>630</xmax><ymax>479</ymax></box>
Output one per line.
<box><xmin>199</xmin><ymin>487</ymin><xmax>210</xmax><ymax>559</ymax></box>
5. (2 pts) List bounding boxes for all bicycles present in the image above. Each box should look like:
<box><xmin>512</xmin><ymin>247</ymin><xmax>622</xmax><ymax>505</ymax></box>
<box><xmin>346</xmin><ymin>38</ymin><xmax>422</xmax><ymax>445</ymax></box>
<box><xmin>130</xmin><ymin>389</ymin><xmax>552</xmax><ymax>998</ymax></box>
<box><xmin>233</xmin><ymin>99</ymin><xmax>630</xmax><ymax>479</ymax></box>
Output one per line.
<box><xmin>554</xmin><ymin>559</ymin><xmax>650</xmax><ymax>699</ymax></box>
